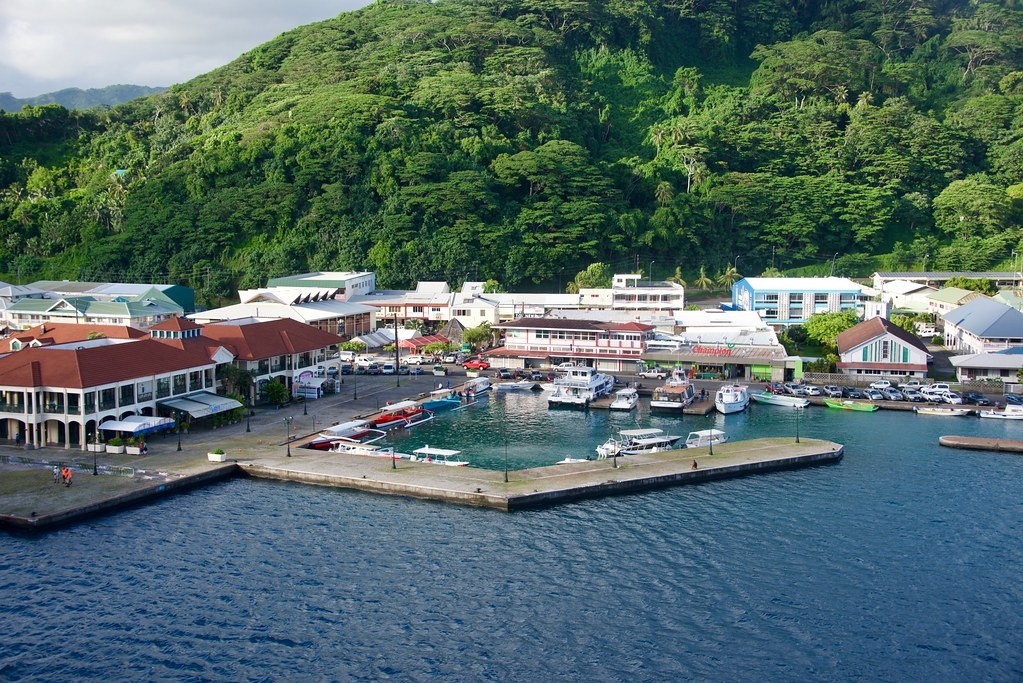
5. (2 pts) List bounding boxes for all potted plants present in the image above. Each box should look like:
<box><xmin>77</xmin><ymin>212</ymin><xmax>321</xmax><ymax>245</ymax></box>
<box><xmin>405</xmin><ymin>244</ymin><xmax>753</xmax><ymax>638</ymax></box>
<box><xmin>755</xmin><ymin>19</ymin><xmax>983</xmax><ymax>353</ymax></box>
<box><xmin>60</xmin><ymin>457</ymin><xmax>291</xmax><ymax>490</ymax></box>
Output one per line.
<box><xmin>210</xmin><ymin>417</ymin><xmax>218</xmax><ymax>429</ymax></box>
<box><xmin>231</xmin><ymin>410</ymin><xmax>239</xmax><ymax>424</ymax></box>
<box><xmin>181</xmin><ymin>422</ymin><xmax>189</xmax><ymax>433</ymax></box>
<box><xmin>216</xmin><ymin>415</ymin><xmax>224</xmax><ymax>426</ymax></box>
<box><xmin>126</xmin><ymin>438</ymin><xmax>141</xmax><ymax>456</ymax></box>
<box><xmin>466</xmin><ymin>368</ymin><xmax>479</xmax><ymax>378</ymax></box>
<box><xmin>87</xmin><ymin>432</ymin><xmax>105</xmax><ymax>452</ymax></box>
<box><xmin>175</xmin><ymin>425</ymin><xmax>179</xmax><ymax>434</ymax></box>
<box><xmin>433</xmin><ymin>367</ymin><xmax>446</xmax><ymax>376</ymax></box>
<box><xmin>207</xmin><ymin>448</ymin><xmax>227</xmax><ymax>463</ymax></box>
<box><xmin>106</xmin><ymin>437</ymin><xmax>124</xmax><ymax>454</ymax></box>
<box><xmin>225</xmin><ymin>412</ymin><xmax>232</xmax><ymax>425</ymax></box>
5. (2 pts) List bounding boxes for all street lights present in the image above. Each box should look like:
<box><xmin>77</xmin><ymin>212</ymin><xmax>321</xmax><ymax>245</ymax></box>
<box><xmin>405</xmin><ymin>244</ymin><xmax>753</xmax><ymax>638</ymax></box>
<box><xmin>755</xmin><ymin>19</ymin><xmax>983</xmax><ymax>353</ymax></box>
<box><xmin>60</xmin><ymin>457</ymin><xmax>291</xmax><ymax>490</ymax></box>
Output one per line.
<box><xmin>243</xmin><ymin>396</ymin><xmax>253</xmax><ymax>432</ymax></box>
<box><xmin>172</xmin><ymin>410</ymin><xmax>183</xmax><ymax>450</ymax></box>
<box><xmin>793</xmin><ymin>404</ymin><xmax>802</xmax><ymax>443</ymax></box>
<box><xmin>706</xmin><ymin>415</ymin><xmax>716</xmax><ymax>455</ymax></box>
<box><xmin>300</xmin><ymin>381</ymin><xmax>311</xmax><ymax>415</ymax></box>
<box><xmin>609</xmin><ymin>425</ymin><xmax>620</xmax><ymax>467</ymax></box>
<box><xmin>353</xmin><ymin>371</ymin><xmax>357</xmax><ymax>400</ymax></box>
<box><xmin>388</xmin><ymin>426</ymin><xmax>398</xmax><ymax>469</ymax></box>
<box><xmin>283</xmin><ymin>416</ymin><xmax>293</xmax><ymax>457</ymax></box>
<box><xmin>88</xmin><ymin>431</ymin><xmax>99</xmax><ymax>476</ymax></box>
<box><xmin>501</xmin><ymin>439</ymin><xmax>510</xmax><ymax>482</ymax></box>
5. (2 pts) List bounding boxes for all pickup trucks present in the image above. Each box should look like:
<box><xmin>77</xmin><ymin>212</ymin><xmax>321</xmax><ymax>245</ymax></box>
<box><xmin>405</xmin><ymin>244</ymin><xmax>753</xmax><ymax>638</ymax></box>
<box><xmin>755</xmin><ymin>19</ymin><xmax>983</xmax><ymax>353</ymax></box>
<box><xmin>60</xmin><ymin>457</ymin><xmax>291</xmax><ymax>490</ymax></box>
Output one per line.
<box><xmin>917</xmin><ymin>329</ymin><xmax>940</xmax><ymax>338</ymax></box>
<box><xmin>639</xmin><ymin>369</ymin><xmax>667</xmax><ymax>380</ymax></box>
<box><xmin>898</xmin><ymin>380</ymin><xmax>929</xmax><ymax>391</ymax></box>
<box><xmin>462</xmin><ymin>360</ymin><xmax>490</xmax><ymax>370</ymax></box>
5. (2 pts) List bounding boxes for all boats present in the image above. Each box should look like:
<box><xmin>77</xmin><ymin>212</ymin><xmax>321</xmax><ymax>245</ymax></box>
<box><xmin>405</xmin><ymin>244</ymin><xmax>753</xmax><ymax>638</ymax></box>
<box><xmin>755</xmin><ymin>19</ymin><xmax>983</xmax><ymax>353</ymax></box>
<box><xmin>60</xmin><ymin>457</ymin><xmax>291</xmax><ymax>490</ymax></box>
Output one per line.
<box><xmin>912</xmin><ymin>406</ymin><xmax>971</xmax><ymax>416</ymax></box>
<box><xmin>556</xmin><ymin>454</ymin><xmax>591</xmax><ymax>465</ymax></box>
<box><xmin>975</xmin><ymin>405</ymin><xmax>1023</xmax><ymax>419</ymax></box>
<box><xmin>650</xmin><ymin>370</ymin><xmax>696</xmax><ymax>414</ymax></box>
<box><xmin>422</xmin><ymin>377</ymin><xmax>557</xmax><ymax>413</ymax></box>
<box><xmin>714</xmin><ymin>383</ymin><xmax>749</xmax><ymax>413</ymax></box>
<box><xmin>548</xmin><ymin>367</ymin><xmax>614</xmax><ymax>408</ymax></box>
<box><xmin>678</xmin><ymin>429</ymin><xmax>730</xmax><ymax>449</ymax></box>
<box><xmin>374</xmin><ymin>400</ymin><xmax>423</xmax><ymax>429</ymax></box>
<box><xmin>310</xmin><ymin>420</ymin><xmax>410</xmax><ymax>460</ymax></box>
<box><xmin>751</xmin><ymin>392</ymin><xmax>810</xmax><ymax>407</ymax></box>
<box><xmin>595</xmin><ymin>428</ymin><xmax>683</xmax><ymax>456</ymax></box>
<box><xmin>609</xmin><ymin>388</ymin><xmax>639</xmax><ymax>411</ymax></box>
<box><xmin>823</xmin><ymin>398</ymin><xmax>879</xmax><ymax>412</ymax></box>
<box><xmin>410</xmin><ymin>444</ymin><xmax>469</xmax><ymax>466</ymax></box>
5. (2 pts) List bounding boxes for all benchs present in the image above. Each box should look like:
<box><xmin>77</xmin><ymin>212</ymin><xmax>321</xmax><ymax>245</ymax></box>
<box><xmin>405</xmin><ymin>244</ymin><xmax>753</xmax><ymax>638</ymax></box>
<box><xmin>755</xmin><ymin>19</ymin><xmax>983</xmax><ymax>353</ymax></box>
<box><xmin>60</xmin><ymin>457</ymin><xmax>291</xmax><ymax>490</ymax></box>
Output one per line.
<box><xmin>419</xmin><ymin>392</ymin><xmax>426</xmax><ymax>398</ymax></box>
<box><xmin>288</xmin><ymin>434</ymin><xmax>297</xmax><ymax>440</ymax></box>
<box><xmin>353</xmin><ymin>414</ymin><xmax>361</xmax><ymax>419</ymax></box>
<box><xmin>332</xmin><ymin>421</ymin><xmax>340</xmax><ymax>426</ymax></box>
<box><xmin>402</xmin><ymin>397</ymin><xmax>410</xmax><ymax>402</ymax></box>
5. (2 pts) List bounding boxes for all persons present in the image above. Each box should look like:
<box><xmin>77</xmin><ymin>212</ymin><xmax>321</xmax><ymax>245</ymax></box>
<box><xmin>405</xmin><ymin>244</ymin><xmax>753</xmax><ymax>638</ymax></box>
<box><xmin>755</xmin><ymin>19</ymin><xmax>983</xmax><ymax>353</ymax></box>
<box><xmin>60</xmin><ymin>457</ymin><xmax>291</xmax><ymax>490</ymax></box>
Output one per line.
<box><xmin>444</xmin><ymin>367</ymin><xmax>448</xmax><ymax>378</ymax></box>
<box><xmin>691</xmin><ymin>459</ymin><xmax>697</xmax><ymax>469</ymax></box>
<box><xmin>16</xmin><ymin>433</ymin><xmax>20</xmax><ymax>447</ymax></box>
<box><xmin>698</xmin><ymin>388</ymin><xmax>709</xmax><ymax>401</ymax></box>
<box><xmin>52</xmin><ymin>466</ymin><xmax>60</xmax><ymax>484</ymax></box>
<box><xmin>625</xmin><ymin>380</ymin><xmax>629</xmax><ymax>388</ymax></box>
<box><xmin>61</xmin><ymin>467</ymin><xmax>73</xmax><ymax>487</ymax></box>
<box><xmin>144</xmin><ymin>441</ymin><xmax>148</xmax><ymax>455</ymax></box>
<box><xmin>497</xmin><ymin>363</ymin><xmax>542</xmax><ymax>382</ymax></box>
<box><xmin>446</xmin><ymin>379</ymin><xmax>449</xmax><ymax>389</ymax></box>
<box><xmin>139</xmin><ymin>441</ymin><xmax>144</xmax><ymax>455</ymax></box>
<box><xmin>438</xmin><ymin>381</ymin><xmax>443</xmax><ymax>388</ymax></box>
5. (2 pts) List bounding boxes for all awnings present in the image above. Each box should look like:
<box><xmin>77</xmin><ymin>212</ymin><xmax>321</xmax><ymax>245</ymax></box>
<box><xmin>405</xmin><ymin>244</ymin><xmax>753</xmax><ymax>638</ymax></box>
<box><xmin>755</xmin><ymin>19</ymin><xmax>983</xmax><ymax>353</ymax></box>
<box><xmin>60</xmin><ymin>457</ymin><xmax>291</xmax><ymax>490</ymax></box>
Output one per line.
<box><xmin>156</xmin><ymin>391</ymin><xmax>243</xmax><ymax>418</ymax></box>
<box><xmin>122</xmin><ymin>415</ymin><xmax>175</xmax><ymax>431</ymax></box>
<box><xmin>98</xmin><ymin>420</ymin><xmax>154</xmax><ymax>437</ymax></box>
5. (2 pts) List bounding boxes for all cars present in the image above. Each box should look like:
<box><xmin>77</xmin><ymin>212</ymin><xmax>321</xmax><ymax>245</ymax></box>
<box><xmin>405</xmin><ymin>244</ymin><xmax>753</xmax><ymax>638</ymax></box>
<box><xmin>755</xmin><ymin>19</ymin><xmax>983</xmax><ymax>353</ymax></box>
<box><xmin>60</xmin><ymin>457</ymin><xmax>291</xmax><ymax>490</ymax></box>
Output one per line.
<box><xmin>842</xmin><ymin>386</ymin><xmax>860</xmax><ymax>399</ymax></box>
<box><xmin>499</xmin><ymin>369</ymin><xmax>510</xmax><ymax>378</ymax></box>
<box><xmin>314</xmin><ymin>350</ymin><xmax>471</xmax><ymax>377</ymax></box>
<box><xmin>1007</xmin><ymin>394</ymin><xmax>1023</xmax><ymax>405</ymax></box>
<box><xmin>530</xmin><ymin>370</ymin><xmax>542</xmax><ymax>380</ymax></box>
<box><xmin>553</xmin><ymin>362</ymin><xmax>575</xmax><ymax>372</ymax></box>
<box><xmin>920</xmin><ymin>388</ymin><xmax>942</xmax><ymax>402</ymax></box>
<box><xmin>513</xmin><ymin>370</ymin><xmax>525</xmax><ymax>379</ymax></box>
<box><xmin>765</xmin><ymin>382</ymin><xmax>785</xmax><ymax>395</ymax></box>
<box><xmin>784</xmin><ymin>383</ymin><xmax>804</xmax><ymax>395</ymax></box>
<box><xmin>803</xmin><ymin>386</ymin><xmax>820</xmax><ymax>395</ymax></box>
<box><xmin>822</xmin><ymin>385</ymin><xmax>842</xmax><ymax>398</ymax></box>
<box><xmin>546</xmin><ymin>372</ymin><xmax>556</xmax><ymax>382</ymax></box>
<box><xmin>863</xmin><ymin>388</ymin><xmax>883</xmax><ymax>400</ymax></box>
<box><xmin>942</xmin><ymin>392</ymin><xmax>962</xmax><ymax>405</ymax></box>
<box><xmin>928</xmin><ymin>383</ymin><xmax>950</xmax><ymax>394</ymax></box>
<box><xmin>869</xmin><ymin>379</ymin><xmax>893</xmax><ymax>389</ymax></box>
<box><xmin>902</xmin><ymin>389</ymin><xmax>922</xmax><ymax>402</ymax></box>
<box><xmin>927</xmin><ymin>356</ymin><xmax>934</xmax><ymax>363</ymax></box>
<box><xmin>962</xmin><ymin>392</ymin><xmax>991</xmax><ymax>406</ymax></box>
<box><xmin>878</xmin><ymin>387</ymin><xmax>903</xmax><ymax>401</ymax></box>
